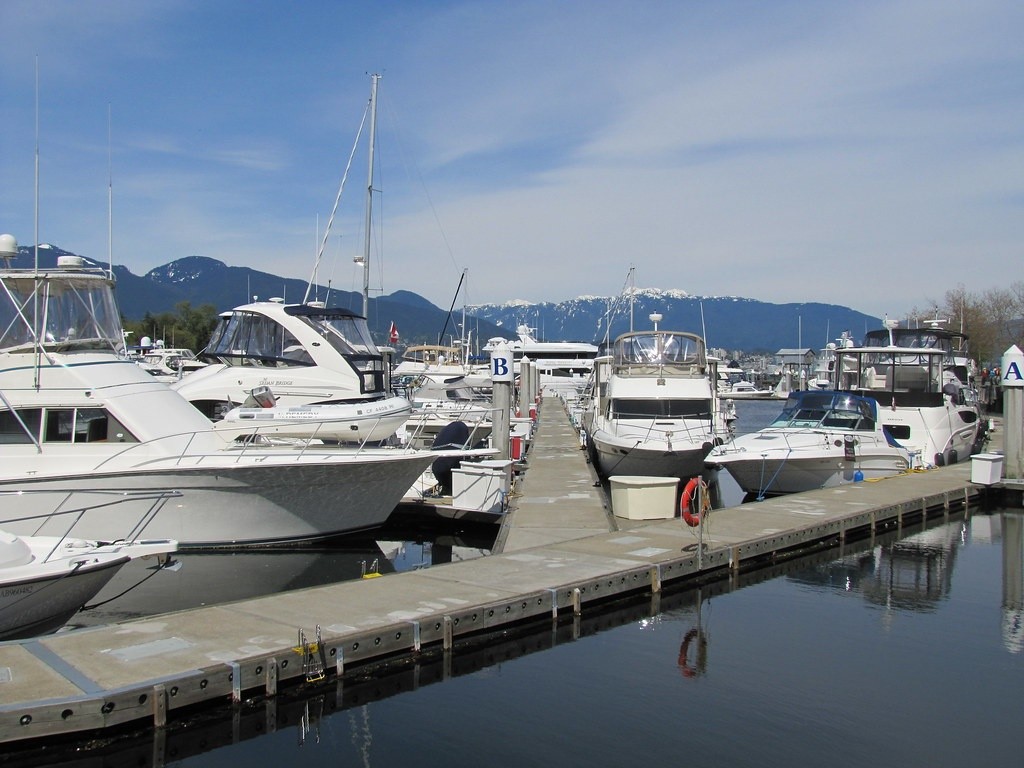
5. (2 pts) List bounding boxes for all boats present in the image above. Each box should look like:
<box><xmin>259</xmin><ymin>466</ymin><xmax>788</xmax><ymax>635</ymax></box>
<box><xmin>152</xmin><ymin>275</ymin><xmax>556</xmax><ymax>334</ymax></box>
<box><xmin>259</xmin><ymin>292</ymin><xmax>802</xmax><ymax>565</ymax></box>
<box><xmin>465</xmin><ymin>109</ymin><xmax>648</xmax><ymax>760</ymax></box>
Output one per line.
<box><xmin>0</xmin><ymin>70</ymin><xmax>1001</xmax><ymax>642</ymax></box>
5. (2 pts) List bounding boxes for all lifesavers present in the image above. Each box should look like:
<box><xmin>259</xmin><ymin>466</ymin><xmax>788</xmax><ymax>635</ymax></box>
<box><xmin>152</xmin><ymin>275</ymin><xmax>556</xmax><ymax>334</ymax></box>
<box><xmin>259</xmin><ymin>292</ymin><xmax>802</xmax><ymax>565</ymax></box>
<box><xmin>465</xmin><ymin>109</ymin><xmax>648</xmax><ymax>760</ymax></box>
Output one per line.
<box><xmin>678</xmin><ymin>629</ymin><xmax>708</xmax><ymax>677</ymax></box>
<box><xmin>680</xmin><ymin>478</ymin><xmax>709</xmax><ymax>526</ymax></box>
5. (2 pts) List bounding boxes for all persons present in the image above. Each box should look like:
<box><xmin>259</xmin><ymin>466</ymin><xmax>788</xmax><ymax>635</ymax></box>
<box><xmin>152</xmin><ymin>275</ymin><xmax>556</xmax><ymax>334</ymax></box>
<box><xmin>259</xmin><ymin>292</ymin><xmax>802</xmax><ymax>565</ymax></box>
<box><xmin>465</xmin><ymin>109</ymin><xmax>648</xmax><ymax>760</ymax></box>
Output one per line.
<box><xmin>981</xmin><ymin>366</ymin><xmax>999</xmax><ymax>381</ymax></box>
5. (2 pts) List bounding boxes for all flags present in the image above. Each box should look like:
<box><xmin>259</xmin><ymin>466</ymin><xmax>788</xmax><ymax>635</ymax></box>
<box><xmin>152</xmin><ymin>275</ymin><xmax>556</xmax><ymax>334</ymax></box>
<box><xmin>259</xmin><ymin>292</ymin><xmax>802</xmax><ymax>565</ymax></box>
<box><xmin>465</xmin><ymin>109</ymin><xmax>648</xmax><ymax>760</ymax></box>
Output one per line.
<box><xmin>389</xmin><ymin>323</ymin><xmax>399</xmax><ymax>343</ymax></box>
